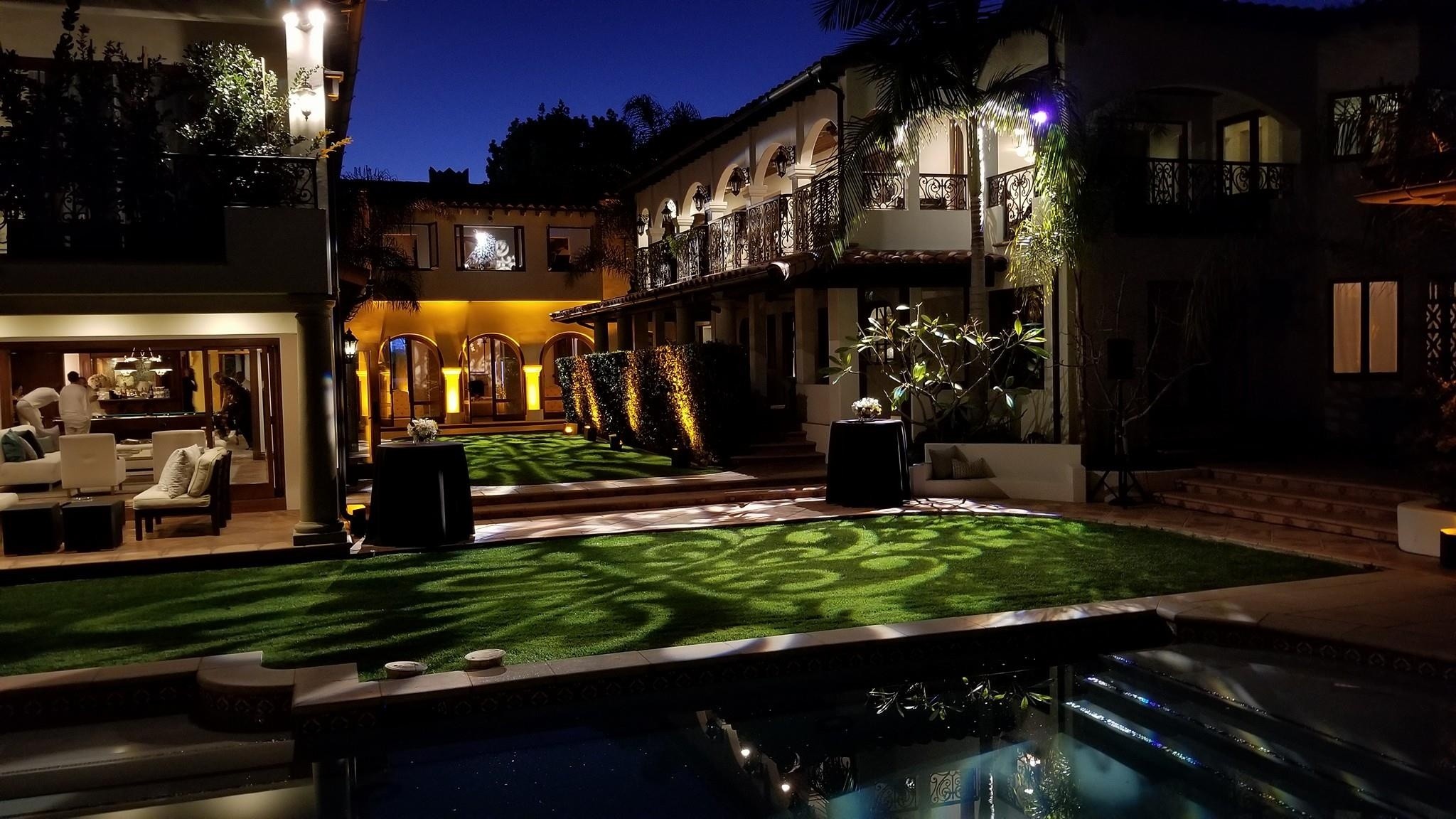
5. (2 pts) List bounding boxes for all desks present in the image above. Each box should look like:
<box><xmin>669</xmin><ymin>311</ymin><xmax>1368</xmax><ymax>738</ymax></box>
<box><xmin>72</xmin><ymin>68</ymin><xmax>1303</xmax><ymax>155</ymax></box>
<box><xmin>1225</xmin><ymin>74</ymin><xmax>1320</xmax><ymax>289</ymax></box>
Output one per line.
<box><xmin>367</xmin><ymin>441</ymin><xmax>475</xmax><ymax>548</ymax></box>
<box><xmin>825</xmin><ymin>418</ymin><xmax>910</xmax><ymax>509</ymax></box>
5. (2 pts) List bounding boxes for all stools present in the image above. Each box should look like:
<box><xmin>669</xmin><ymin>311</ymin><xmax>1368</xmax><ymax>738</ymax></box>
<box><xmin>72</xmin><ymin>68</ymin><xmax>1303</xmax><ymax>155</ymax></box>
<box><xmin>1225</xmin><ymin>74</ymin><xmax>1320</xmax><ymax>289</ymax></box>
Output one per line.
<box><xmin>0</xmin><ymin>497</ymin><xmax>125</xmax><ymax>555</ymax></box>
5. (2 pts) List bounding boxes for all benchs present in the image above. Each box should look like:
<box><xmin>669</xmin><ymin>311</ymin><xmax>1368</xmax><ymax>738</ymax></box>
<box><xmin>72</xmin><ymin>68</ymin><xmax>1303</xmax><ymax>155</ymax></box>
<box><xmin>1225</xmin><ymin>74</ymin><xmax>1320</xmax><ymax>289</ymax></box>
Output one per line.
<box><xmin>0</xmin><ymin>424</ymin><xmax>62</xmax><ymax>492</ymax></box>
<box><xmin>132</xmin><ymin>447</ymin><xmax>232</xmax><ymax>541</ymax></box>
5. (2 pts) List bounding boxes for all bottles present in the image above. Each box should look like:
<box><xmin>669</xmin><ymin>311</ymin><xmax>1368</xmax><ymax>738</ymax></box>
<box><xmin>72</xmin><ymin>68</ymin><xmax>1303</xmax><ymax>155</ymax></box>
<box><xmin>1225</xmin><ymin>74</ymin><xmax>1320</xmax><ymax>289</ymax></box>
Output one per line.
<box><xmin>149</xmin><ymin>385</ymin><xmax>154</xmax><ymax>398</ymax></box>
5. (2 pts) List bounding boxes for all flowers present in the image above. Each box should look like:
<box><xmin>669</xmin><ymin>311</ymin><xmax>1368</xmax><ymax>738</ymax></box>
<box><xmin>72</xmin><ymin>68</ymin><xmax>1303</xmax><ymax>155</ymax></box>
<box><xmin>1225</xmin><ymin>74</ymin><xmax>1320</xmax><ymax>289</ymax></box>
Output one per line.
<box><xmin>852</xmin><ymin>395</ymin><xmax>884</xmax><ymax>417</ymax></box>
<box><xmin>406</xmin><ymin>416</ymin><xmax>440</xmax><ymax>443</ymax></box>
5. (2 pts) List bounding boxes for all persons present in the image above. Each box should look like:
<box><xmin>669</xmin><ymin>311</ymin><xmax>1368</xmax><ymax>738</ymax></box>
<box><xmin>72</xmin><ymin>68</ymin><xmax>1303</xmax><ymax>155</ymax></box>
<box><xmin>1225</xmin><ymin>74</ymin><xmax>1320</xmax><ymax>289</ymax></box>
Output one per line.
<box><xmin>13</xmin><ymin>382</ymin><xmax>23</xmax><ymax>421</ymax></box>
<box><xmin>59</xmin><ymin>371</ymin><xmax>91</xmax><ymax>434</ymax></box>
<box><xmin>235</xmin><ymin>371</ymin><xmax>250</xmax><ymax>390</ymax></box>
<box><xmin>80</xmin><ymin>378</ymin><xmax>100</xmax><ymax>411</ymax></box>
<box><xmin>16</xmin><ymin>387</ymin><xmax>59</xmax><ymax>429</ymax></box>
<box><xmin>213</xmin><ymin>373</ymin><xmax>253</xmax><ymax>450</ymax></box>
<box><xmin>182</xmin><ymin>367</ymin><xmax>198</xmax><ymax>411</ymax></box>
<box><xmin>225</xmin><ymin>368</ymin><xmax>235</xmax><ymax>377</ymax></box>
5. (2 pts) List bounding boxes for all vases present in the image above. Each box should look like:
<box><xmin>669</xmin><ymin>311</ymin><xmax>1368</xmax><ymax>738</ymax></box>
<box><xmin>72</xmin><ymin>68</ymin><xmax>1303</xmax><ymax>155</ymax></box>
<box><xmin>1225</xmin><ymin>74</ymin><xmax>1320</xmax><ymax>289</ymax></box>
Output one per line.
<box><xmin>414</xmin><ymin>432</ymin><xmax>436</xmax><ymax>444</ymax></box>
<box><xmin>858</xmin><ymin>408</ymin><xmax>875</xmax><ymax>423</ymax></box>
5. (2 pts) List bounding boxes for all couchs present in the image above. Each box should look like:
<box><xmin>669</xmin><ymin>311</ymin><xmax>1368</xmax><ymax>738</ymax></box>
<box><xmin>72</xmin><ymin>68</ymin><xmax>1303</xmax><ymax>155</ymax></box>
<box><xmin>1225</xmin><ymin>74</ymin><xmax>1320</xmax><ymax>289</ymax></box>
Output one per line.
<box><xmin>907</xmin><ymin>442</ymin><xmax>1087</xmax><ymax>502</ymax></box>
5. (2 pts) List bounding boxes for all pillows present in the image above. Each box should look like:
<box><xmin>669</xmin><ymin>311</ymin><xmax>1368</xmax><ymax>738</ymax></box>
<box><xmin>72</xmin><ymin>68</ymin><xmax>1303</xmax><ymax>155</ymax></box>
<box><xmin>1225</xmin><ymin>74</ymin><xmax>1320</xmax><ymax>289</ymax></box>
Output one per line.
<box><xmin>1</xmin><ymin>425</ymin><xmax>60</xmax><ymax>461</ymax></box>
<box><xmin>159</xmin><ymin>442</ymin><xmax>204</xmax><ymax>498</ymax></box>
<box><xmin>929</xmin><ymin>445</ymin><xmax>988</xmax><ymax>480</ymax></box>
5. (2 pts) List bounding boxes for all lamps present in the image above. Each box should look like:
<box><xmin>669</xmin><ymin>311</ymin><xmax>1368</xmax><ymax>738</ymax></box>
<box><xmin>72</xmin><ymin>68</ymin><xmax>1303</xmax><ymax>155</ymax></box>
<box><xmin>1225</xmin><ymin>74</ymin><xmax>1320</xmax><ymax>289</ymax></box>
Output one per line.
<box><xmin>563</xmin><ymin>422</ymin><xmax>691</xmax><ymax>467</ymax></box>
<box><xmin>770</xmin><ymin>144</ymin><xmax>797</xmax><ymax>178</ymax></box>
<box><xmin>115</xmin><ymin>348</ymin><xmax>174</xmax><ymax>377</ymax></box>
<box><xmin>296</xmin><ymin>70</ymin><xmax>345</xmax><ymax>122</ymax></box>
<box><xmin>767</xmin><ymin>259</ymin><xmax>792</xmax><ymax>282</ymax></box>
<box><xmin>384</xmin><ymin>649</ymin><xmax>506</xmax><ymax>679</ymax></box>
<box><xmin>728</xmin><ymin>166</ymin><xmax>750</xmax><ymax>197</ymax></box>
<box><xmin>636</xmin><ymin>183</ymin><xmax>711</xmax><ymax>236</ymax></box>
<box><xmin>344</xmin><ymin>328</ymin><xmax>360</xmax><ymax>365</ymax></box>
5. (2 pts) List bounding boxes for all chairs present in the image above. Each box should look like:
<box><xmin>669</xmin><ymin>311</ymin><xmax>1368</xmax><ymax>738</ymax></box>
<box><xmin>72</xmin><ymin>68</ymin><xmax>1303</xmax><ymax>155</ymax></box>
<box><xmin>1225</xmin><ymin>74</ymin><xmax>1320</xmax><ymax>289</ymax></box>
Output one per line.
<box><xmin>57</xmin><ymin>430</ymin><xmax>127</xmax><ymax>498</ymax></box>
<box><xmin>152</xmin><ymin>430</ymin><xmax>206</xmax><ymax>482</ymax></box>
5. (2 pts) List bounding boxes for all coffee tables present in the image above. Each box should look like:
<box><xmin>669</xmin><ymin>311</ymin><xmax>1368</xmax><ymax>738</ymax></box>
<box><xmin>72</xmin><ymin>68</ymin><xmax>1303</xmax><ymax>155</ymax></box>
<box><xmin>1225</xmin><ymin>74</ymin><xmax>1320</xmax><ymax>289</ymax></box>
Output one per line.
<box><xmin>115</xmin><ymin>442</ymin><xmax>153</xmax><ymax>476</ymax></box>
